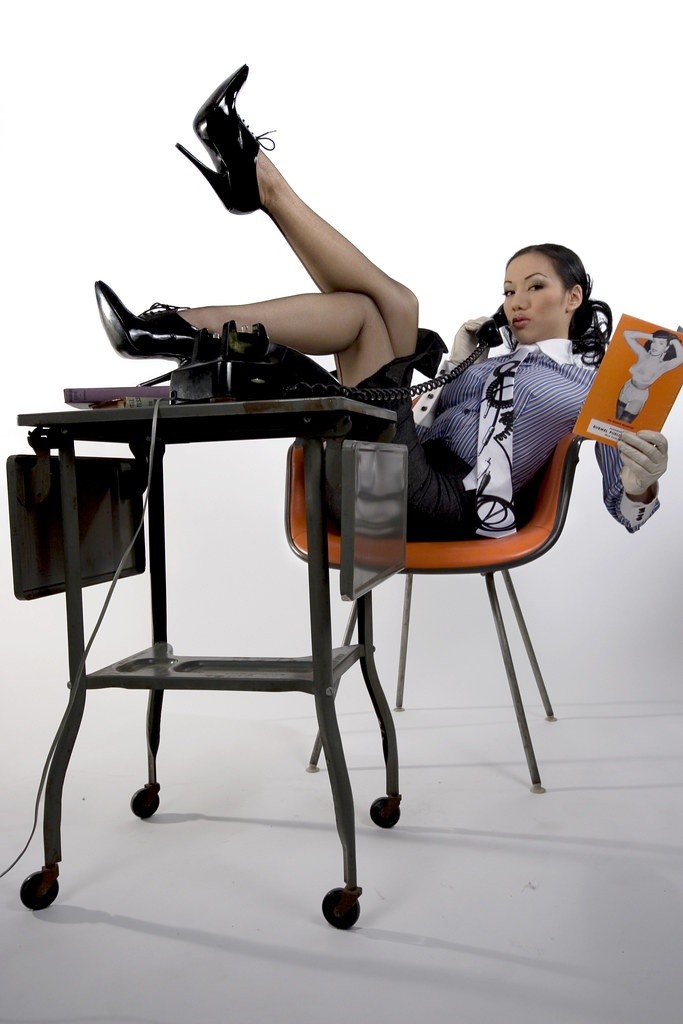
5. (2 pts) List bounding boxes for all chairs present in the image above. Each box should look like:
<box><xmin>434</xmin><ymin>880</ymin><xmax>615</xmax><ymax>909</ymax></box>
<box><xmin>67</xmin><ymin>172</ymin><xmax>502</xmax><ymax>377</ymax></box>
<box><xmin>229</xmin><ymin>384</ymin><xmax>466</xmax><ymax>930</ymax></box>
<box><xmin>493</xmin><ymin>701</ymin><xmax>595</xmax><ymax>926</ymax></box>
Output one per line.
<box><xmin>285</xmin><ymin>396</ymin><xmax>586</xmax><ymax>795</ymax></box>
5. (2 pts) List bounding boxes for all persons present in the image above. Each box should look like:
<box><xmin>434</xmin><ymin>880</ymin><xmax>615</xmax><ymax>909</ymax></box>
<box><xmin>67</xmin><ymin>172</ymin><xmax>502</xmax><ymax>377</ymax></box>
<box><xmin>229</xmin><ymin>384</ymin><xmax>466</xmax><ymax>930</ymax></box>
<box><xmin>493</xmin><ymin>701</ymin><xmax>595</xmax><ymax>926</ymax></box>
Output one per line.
<box><xmin>95</xmin><ymin>62</ymin><xmax>669</xmax><ymax>542</ymax></box>
<box><xmin>615</xmin><ymin>328</ymin><xmax>683</xmax><ymax>425</ymax></box>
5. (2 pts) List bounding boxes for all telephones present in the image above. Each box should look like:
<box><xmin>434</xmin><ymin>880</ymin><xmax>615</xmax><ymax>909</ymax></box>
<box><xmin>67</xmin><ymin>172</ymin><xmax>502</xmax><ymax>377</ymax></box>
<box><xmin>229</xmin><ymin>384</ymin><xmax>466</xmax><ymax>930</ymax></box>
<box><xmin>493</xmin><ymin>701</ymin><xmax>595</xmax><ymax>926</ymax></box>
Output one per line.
<box><xmin>477</xmin><ymin>303</ymin><xmax>511</xmax><ymax>348</ymax></box>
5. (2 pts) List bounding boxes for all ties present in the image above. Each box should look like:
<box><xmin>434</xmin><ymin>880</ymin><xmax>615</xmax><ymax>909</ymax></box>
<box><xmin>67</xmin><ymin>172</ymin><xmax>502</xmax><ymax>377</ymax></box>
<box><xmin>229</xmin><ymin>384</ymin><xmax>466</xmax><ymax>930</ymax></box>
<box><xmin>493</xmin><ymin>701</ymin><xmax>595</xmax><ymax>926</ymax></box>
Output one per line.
<box><xmin>463</xmin><ymin>347</ymin><xmax>531</xmax><ymax>541</ymax></box>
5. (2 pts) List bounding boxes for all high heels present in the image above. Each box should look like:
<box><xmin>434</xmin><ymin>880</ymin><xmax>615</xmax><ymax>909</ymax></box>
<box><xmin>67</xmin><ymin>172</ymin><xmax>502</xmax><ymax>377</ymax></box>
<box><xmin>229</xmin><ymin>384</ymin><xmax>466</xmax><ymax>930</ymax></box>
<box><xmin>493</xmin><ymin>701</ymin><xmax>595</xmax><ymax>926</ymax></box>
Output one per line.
<box><xmin>174</xmin><ymin>64</ymin><xmax>277</xmax><ymax>216</ymax></box>
<box><xmin>95</xmin><ymin>280</ymin><xmax>221</xmax><ymax>386</ymax></box>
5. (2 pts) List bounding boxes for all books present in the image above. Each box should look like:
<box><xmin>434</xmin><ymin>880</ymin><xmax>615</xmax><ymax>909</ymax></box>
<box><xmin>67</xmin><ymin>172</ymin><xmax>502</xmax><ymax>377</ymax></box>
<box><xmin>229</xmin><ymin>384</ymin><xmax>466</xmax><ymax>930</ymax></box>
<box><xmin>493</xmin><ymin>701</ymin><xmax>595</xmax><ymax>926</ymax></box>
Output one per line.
<box><xmin>573</xmin><ymin>313</ymin><xmax>683</xmax><ymax>449</ymax></box>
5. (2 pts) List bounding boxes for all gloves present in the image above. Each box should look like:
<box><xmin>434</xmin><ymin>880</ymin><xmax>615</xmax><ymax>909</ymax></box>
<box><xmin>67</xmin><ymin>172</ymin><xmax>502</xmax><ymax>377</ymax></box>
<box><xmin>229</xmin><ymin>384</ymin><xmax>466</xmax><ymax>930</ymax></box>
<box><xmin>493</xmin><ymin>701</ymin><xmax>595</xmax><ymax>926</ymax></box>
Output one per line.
<box><xmin>450</xmin><ymin>316</ymin><xmax>493</xmax><ymax>368</ymax></box>
<box><xmin>617</xmin><ymin>429</ymin><xmax>668</xmax><ymax>495</ymax></box>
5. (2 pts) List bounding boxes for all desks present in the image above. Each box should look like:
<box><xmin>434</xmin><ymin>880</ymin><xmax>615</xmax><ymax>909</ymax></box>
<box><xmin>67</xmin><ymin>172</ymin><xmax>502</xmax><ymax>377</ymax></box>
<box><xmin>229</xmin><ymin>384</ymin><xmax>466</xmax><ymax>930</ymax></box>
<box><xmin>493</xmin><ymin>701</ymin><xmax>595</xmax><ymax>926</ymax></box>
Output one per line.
<box><xmin>5</xmin><ymin>396</ymin><xmax>409</xmax><ymax>930</ymax></box>
